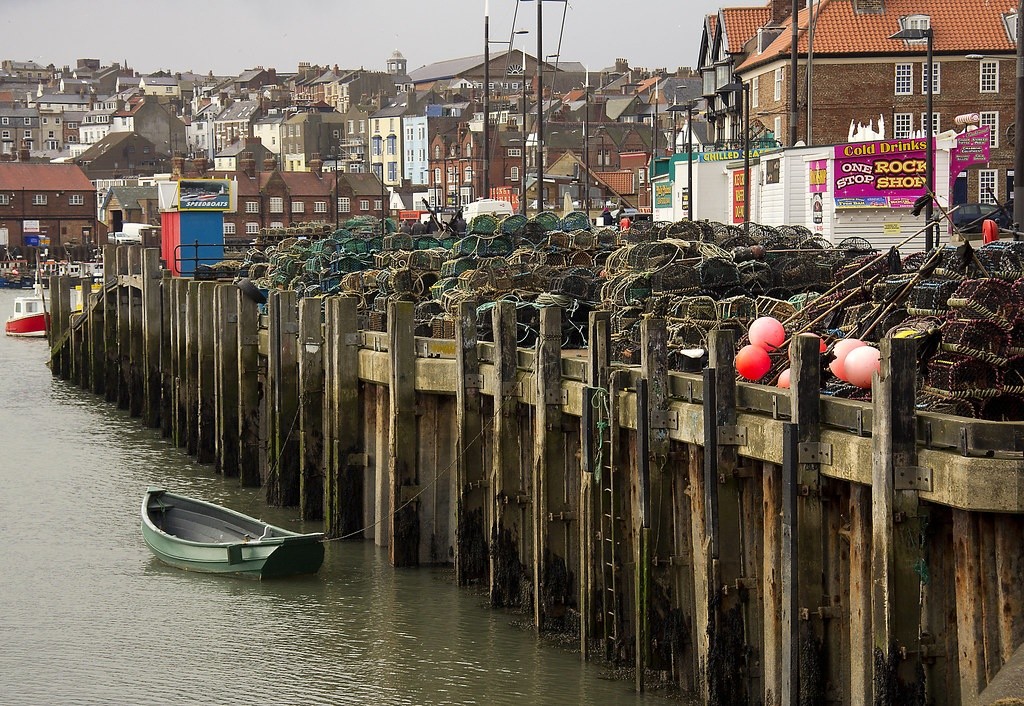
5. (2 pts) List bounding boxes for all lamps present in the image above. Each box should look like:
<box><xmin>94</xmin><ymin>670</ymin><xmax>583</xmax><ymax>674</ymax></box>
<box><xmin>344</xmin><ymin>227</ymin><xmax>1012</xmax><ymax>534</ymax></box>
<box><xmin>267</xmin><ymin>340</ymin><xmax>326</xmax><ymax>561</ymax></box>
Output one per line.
<box><xmin>739</xmin><ymin>119</ymin><xmax>774</xmax><ymax>140</ymax></box>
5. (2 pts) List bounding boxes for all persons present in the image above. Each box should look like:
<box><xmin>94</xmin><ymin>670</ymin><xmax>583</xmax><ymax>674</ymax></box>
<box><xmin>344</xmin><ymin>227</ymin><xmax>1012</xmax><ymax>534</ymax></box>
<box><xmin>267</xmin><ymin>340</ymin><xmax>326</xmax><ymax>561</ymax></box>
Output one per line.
<box><xmin>398</xmin><ymin>212</ymin><xmax>467</xmax><ymax>238</ymax></box>
<box><xmin>600</xmin><ymin>207</ymin><xmax>612</xmax><ymax>225</ymax></box>
<box><xmin>616</xmin><ymin>205</ymin><xmax>626</xmax><ymax>225</ymax></box>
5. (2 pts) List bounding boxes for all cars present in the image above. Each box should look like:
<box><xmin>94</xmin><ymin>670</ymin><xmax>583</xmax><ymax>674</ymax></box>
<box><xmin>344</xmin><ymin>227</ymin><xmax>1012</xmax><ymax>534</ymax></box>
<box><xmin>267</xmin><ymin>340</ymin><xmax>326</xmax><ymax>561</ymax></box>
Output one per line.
<box><xmin>610</xmin><ymin>208</ymin><xmax>639</xmax><ymax>227</ymax></box>
<box><xmin>107</xmin><ymin>231</ymin><xmax>141</xmax><ymax>245</ymax></box>
<box><xmin>953</xmin><ymin>203</ymin><xmax>1012</xmax><ymax>234</ymax></box>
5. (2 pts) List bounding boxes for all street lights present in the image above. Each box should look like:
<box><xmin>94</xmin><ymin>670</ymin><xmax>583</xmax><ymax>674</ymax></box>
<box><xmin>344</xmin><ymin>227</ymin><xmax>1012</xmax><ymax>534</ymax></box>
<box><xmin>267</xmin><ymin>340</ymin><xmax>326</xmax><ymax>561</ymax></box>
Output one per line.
<box><xmin>886</xmin><ymin>26</ymin><xmax>934</xmax><ymax>255</ymax></box>
<box><xmin>717</xmin><ymin>82</ymin><xmax>751</xmax><ymax>235</ymax></box>
<box><xmin>521</xmin><ymin>40</ymin><xmax>561</xmax><ymax>220</ymax></box>
<box><xmin>665</xmin><ymin>104</ymin><xmax>695</xmax><ymax>222</ymax></box>
<box><xmin>321</xmin><ymin>155</ymin><xmax>340</xmax><ymax>230</ymax></box>
<box><xmin>425</xmin><ymin>169</ymin><xmax>437</xmax><ymax>217</ymax></box>
<box><xmin>581</xmin><ymin>64</ymin><xmax>606</xmax><ymax>222</ymax></box>
<box><xmin>673</xmin><ymin>85</ymin><xmax>688</xmax><ymax>154</ymax></box>
<box><xmin>443</xmin><ymin>157</ymin><xmax>469</xmax><ymax>207</ymax></box>
<box><xmin>371</xmin><ymin>162</ymin><xmax>385</xmax><ymax>236</ymax></box>
<box><xmin>964</xmin><ymin>52</ymin><xmax>1023</xmax><ymax>241</ymax></box>
<box><xmin>482</xmin><ymin>1</ymin><xmax>529</xmax><ymax>194</ymax></box>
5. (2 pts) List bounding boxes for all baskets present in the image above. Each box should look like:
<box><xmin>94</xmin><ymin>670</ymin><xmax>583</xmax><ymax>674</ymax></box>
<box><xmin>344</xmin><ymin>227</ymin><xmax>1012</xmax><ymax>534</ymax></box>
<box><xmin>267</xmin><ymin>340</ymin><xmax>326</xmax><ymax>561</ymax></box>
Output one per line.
<box><xmin>193</xmin><ymin>194</ymin><xmax>1024</xmax><ymax>425</ymax></box>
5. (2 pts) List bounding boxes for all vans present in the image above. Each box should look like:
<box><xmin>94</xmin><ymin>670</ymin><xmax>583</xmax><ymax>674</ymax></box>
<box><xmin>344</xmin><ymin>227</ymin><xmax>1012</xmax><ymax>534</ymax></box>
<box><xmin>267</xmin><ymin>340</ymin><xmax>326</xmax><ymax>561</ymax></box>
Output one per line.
<box><xmin>460</xmin><ymin>198</ymin><xmax>514</xmax><ymax>226</ymax></box>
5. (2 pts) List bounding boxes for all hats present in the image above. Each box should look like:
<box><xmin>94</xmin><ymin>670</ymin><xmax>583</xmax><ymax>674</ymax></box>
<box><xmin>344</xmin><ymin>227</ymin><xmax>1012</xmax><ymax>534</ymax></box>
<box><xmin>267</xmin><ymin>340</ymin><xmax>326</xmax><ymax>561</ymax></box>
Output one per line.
<box><xmin>604</xmin><ymin>208</ymin><xmax>608</xmax><ymax>211</ymax></box>
<box><xmin>619</xmin><ymin>205</ymin><xmax>624</xmax><ymax>210</ymax></box>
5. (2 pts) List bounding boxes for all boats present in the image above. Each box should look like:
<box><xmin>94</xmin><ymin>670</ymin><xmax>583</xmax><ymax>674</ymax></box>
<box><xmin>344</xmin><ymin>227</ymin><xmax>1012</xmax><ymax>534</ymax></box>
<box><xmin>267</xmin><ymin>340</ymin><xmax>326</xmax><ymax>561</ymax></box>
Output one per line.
<box><xmin>139</xmin><ymin>484</ymin><xmax>326</xmax><ymax>580</ymax></box>
<box><xmin>0</xmin><ymin>224</ymin><xmax>104</xmax><ymax>289</ymax></box>
<box><xmin>5</xmin><ymin>271</ymin><xmax>50</xmax><ymax>337</ymax></box>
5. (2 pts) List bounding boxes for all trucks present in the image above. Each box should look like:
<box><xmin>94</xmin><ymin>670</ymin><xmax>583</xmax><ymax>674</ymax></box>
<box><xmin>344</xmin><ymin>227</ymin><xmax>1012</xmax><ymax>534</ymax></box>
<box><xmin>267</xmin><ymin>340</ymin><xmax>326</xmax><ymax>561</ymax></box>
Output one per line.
<box><xmin>121</xmin><ymin>223</ymin><xmax>157</xmax><ymax>239</ymax></box>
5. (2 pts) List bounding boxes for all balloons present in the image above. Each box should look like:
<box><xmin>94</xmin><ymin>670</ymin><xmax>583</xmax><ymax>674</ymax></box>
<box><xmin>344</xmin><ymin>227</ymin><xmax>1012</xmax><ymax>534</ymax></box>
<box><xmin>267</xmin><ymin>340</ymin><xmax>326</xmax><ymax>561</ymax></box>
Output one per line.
<box><xmin>734</xmin><ymin>316</ymin><xmax>920</xmax><ymax>389</ymax></box>
